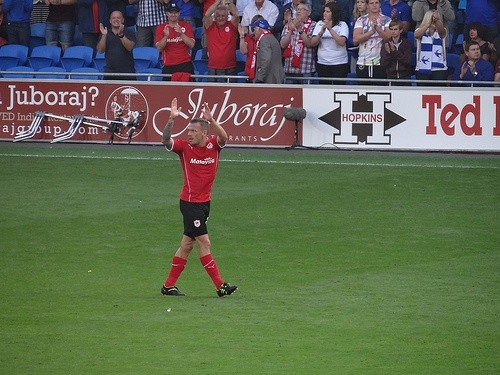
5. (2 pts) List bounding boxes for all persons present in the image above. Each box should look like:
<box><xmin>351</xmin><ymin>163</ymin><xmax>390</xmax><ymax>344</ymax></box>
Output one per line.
<box><xmin>452</xmin><ymin>42</ymin><xmax>494</xmax><ymax>88</ymax></box>
<box><xmin>157</xmin><ymin>96</ymin><xmax>240</xmax><ymax>298</ymax></box>
<box><xmin>153</xmin><ymin>3</ymin><xmax>196</xmax><ymax>83</ymax></box>
<box><xmin>247</xmin><ymin>19</ymin><xmax>286</xmax><ymax>84</ymax></box>
<box><xmin>173</xmin><ymin>0</ymin><xmax>456</xmax><ymax>30</ymax></box>
<box><xmin>414</xmin><ymin>10</ymin><xmax>448</xmax><ymax>85</ymax></box>
<box><xmin>129</xmin><ymin>0</ymin><xmax>174</xmax><ymax>50</ymax></box>
<box><xmin>43</xmin><ymin>0</ymin><xmax>76</xmax><ymax>51</ymax></box>
<box><xmin>237</xmin><ymin>14</ymin><xmax>264</xmax><ymax>84</ymax></box>
<box><xmin>279</xmin><ymin>2</ymin><xmax>319</xmax><ymax>84</ymax></box>
<box><xmin>353</xmin><ymin>0</ymin><xmax>392</xmax><ymax>85</ymax></box>
<box><xmin>381</xmin><ymin>19</ymin><xmax>411</xmax><ymax>87</ymax></box>
<box><xmin>96</xmin><ymin>10</ymin><xmax>137</xmax><ymax>80</ymax></box>
<box><xmin>455</xmin><ymin>22</ymin><xmax>492</xmax><ymax>64</ymax></box>
<box><xmin>4</xmin><ymin>0</ymin><xmax>33</xmax><ymax>55</ymax></box>
<box><xmin>310</xmin><ymin>3</ymin><xmax>349</xmax><ymax>85</ymax></box>
<box><xmin>198</xmin><ymin>0</ymin><xmax>239</xmax><ymax>84</ymax></box>
<box><xmin>81</xmin><ymin>0</ymin><xmax>138</xmax><ymax>46</ymax></box>
<box><xmin>29</xmin><ymin>1</ymin><xmax>52</xmax><ymax>24</ymax></box>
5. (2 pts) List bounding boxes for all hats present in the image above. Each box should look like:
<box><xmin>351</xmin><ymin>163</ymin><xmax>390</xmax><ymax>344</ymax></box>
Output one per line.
<box><xmin>249</xmin><ymin>19</ymin><xmax>271</xmax><ymax>29</ymax></box>
<box><xmin>166</xmin><ymin>3</ymin><xmax>182</xmax><ymax>12</ymax></box>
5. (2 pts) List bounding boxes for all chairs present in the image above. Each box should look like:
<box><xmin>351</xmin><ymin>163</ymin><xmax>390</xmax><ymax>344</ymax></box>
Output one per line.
<box><xmin>0</xmin><ymin>16</ymin><xmax>468</xmax><ymax>85</ymax></box>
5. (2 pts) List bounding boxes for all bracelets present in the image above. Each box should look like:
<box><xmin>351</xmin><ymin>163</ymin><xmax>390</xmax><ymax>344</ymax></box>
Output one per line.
<box><xmin>318</xmin><ymin>31</ymin><xmax>323</xmax><ymax>37</ymax></box>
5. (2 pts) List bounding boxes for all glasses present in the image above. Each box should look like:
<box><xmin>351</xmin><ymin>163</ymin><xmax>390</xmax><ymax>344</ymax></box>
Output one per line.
<box><xmin>295</xmin><ymin>8</ymin><xmax>309</xmax><ymax>12</ymax></box>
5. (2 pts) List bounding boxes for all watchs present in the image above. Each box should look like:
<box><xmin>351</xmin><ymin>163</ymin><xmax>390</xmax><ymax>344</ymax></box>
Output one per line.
<box><xmin>299</xmin><ymin>30</ymin><xmax>304</xmax><ymax>34</ymax></box>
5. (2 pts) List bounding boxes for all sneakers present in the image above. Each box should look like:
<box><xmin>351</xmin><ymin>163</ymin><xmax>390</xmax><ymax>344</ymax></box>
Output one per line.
<box><xmin>215</xmin><ymin>283</ymin><xmax>237</xmax><ymax>298</ymax></box>
<box><xmin>161</xmin><ymin>285</ymin><xmax>186</xmax><ymax>296</ymax></box>
